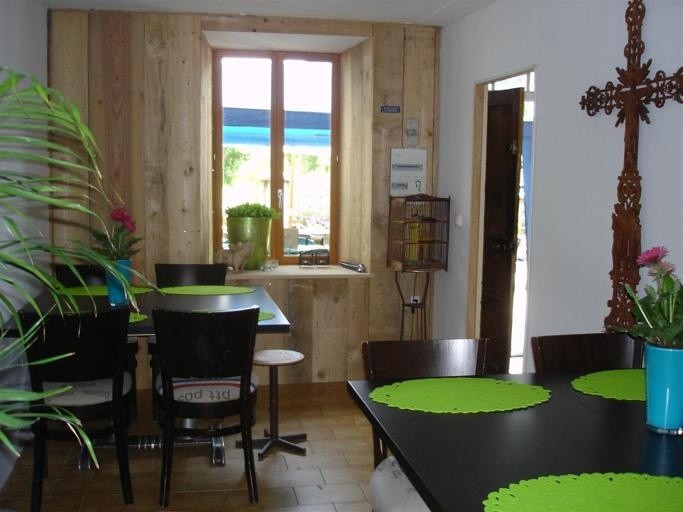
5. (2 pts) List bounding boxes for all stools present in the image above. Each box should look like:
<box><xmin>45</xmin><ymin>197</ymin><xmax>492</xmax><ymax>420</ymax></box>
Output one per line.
<box><xmin>236</xmin><ymin>349</ymin><xmax>307</xmax><ymax>459</ymax></box>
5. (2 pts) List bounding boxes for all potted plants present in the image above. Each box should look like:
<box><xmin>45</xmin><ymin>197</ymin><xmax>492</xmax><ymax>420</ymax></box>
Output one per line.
<box><xmin>224</xmin><ymin>203</ymin><xmax>282</xmax><ymax>270</ymax></box>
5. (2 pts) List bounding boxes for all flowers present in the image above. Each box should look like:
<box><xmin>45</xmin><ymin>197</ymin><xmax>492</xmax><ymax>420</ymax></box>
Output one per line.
<box><xmin>91</xmin><ymin>209</ymin><xmax>144</xmax><ymax>261</ymax></box>
<box><xmin>599</xmin><ymin>246</ymin><xmax>683</xmax><ymax>348</ymax></box>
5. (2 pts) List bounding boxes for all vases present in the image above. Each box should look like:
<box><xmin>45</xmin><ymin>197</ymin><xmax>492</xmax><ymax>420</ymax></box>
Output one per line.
<box><xmin>105</xmin><ymin>259</ymin><xmax>132</xmax><ymax>307</ymax></box>
<box><xmin>643</xmin><ymin>341</ymin><xmax>683</xmax><ymax>437</ymax></box>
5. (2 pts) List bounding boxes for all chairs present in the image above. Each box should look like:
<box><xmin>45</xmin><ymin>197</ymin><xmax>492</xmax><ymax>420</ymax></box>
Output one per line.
<box><xmin>362</xmin><ymin>332</ymin><xmax>636</xmax><ymax>472</ymax></box>
<box><xmin>16</xmin><ymin>307</ymin><xmax>140</xmax><ymax>511</ymax></box>
<box><xmin>154</xmin><ymin>263</ymin><xmax>226</xmax><ymax>288</ymax></box>
<box><xmin>147</xmin><ymin>305</ymin><xmax>261</xmax><ymax>508</ymax></box>
<box><xmin>51</xmin><ymin>263</ymin><xmax>132</xmax><ymax>287</ymax></box>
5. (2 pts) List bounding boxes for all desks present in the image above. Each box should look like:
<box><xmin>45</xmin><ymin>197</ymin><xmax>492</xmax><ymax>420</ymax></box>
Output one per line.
<box><xmin>346</xmin><ymin>369</ymin><xmax>683</xmax><ymax>512</ymax></box>
<box><xmin>1</xmin><ymin>285</ymin><xmax>289</xmax><ymax>466</ymax></box>
